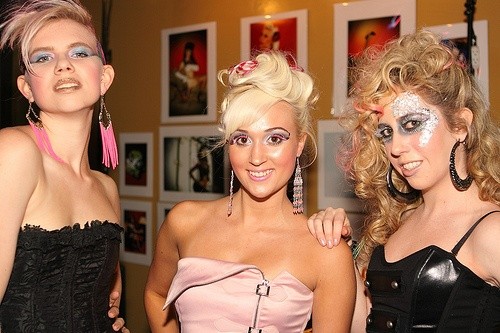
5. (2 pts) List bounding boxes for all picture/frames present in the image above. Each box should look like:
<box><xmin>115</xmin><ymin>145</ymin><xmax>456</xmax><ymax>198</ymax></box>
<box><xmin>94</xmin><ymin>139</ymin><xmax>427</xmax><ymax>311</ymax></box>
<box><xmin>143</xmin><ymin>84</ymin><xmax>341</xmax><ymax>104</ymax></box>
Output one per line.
<box><xmin>422</xmin><ymin>20</ymin><xmax>490</xmax><ymax>110</ymax></box>
<box><xmin>156</xmin><ymin>202</ymin><xmax>175</xmax><ymax>233</ymax></box>
<box><xmin>119</xmin><ymin>132</ymin><xmax>154</xmax><ymax>197</ymax></box>
<box><xmin>239</xmin><ymin>9</ymin><xmax>309</xmax><ymax>73</ymax></box>
<box><xmin>159</xmin><ymin>125</ymin><xmax>230</xmax><ymax>202</ymax></box>
<box><xmin>161</xmin><ymin>22</ymin><xmax>219</xmax><ymax>124</ymax></box>
<box><xmin>117</xmin><ymin>199</ymin><xmax>154</xmax><ymax>265</ymax></box>
<box><xmin>317</xmin><ymin>119</ymin><xmax>364</xmax><ymax>213</ymax></box>
<box><xmin>333</xmin><ymin>0</ymin><xmax>415</xmax><ymax>117</ymax></box>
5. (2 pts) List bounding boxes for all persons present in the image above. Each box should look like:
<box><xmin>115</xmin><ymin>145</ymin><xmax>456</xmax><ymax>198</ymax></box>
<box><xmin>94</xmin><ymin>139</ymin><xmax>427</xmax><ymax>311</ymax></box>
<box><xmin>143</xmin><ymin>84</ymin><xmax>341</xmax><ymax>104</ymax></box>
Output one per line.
<box><xmin>306</xmin><ymin>29</ymin><xmax>500</xmax><ymax>333</ymax></box>
<box><xmin>174</xmin><ymin>43</ymin><xmax>199</xmax><ymax>105</ymax></box>
<box><xmin>257</xmin><ymin>21</ymin><xmax>280</xmax><ymax>52</ymax></box>
<box><xmin>0</xmin><ymin>0</ymin><xmax>131</xmax><ymax>333</ymax></box>
<box><xmin>144</xmin><ymin>51</ymin><xmax>358</xmax><ymax>333</ymax></box>
<box><xmin>189</xmin><ymin>146</ymin><xmax>210</xmax><ymax>193</ymax></box>
<box><xmin>365</xmin><ymin>31</ymin><xmax>374</xmax><ymax>48</ymax></box>
<box><xmin>126</xmin><ymin>150</ymin><xmax>143</xmax><ymax>182</ymax></box>
<box><xmin>124</xmin><ymin>210</ymin><xmax>145</xmax><ymax>253</ymax></box>
